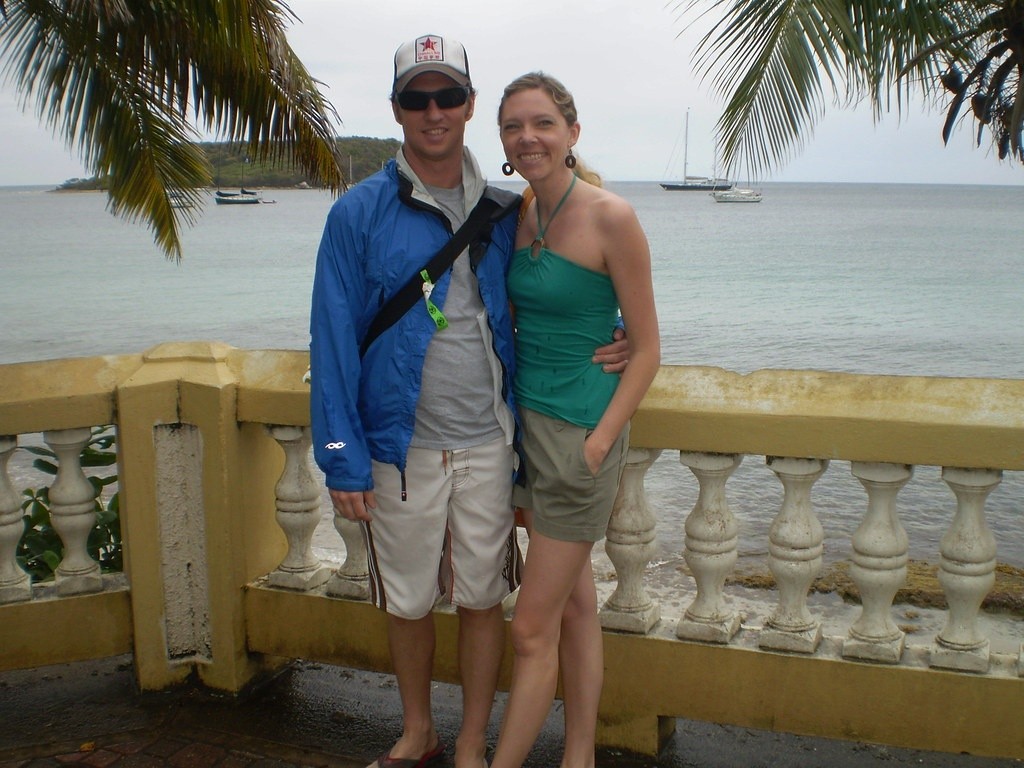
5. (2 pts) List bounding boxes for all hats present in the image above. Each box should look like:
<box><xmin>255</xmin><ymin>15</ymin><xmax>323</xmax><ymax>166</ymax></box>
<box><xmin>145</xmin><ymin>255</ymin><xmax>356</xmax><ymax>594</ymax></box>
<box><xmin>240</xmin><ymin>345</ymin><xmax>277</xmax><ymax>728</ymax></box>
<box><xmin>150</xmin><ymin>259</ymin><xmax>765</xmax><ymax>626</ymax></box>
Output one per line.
<box><xmin>393</xmin><ymin>35</ymin><xmax>473</xmax><ymax>92</ymax></box>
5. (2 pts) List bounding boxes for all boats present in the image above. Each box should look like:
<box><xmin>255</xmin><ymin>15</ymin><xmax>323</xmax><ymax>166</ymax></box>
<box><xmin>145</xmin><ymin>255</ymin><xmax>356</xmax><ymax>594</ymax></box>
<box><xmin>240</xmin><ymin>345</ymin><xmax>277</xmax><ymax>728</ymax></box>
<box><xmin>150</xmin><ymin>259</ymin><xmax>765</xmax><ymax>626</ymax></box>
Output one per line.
<box><xmin>214</xmin><ymin>162</ymin><xmax>262</xmax><ymax>204</ymax></box>
<box><xmin>294</xmin><ymin>181</ymin><xmax>312</xmax><ymax>189</ymax></box>
<box><xmin>712</xmin><ymin>186</ymin><xmax>762</xmax><ymax>203</ymax></box>
<box><xmin>165</xmin><ymin>189</ymin><xmax>194</xmax><ymax>208</ymax></box>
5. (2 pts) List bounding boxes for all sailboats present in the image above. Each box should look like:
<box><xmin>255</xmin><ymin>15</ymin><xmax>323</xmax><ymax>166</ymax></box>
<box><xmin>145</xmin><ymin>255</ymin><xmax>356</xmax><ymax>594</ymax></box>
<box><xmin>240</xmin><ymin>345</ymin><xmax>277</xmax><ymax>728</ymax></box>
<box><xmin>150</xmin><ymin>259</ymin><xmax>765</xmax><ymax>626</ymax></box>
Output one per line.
<box><xmin>658</xmin><ymin>104</ymin><xmax>732</xmax><ymax>191</ymax></box>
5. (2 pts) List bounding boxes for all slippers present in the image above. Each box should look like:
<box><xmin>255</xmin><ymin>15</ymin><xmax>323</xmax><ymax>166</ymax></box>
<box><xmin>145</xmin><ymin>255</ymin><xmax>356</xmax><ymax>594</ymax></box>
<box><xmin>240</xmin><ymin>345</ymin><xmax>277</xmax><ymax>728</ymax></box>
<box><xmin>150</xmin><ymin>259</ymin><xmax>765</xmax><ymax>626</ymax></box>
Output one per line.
<box><xmin>366</xmin><ymin>739</ymin><xmax>445</xmax><ymax>768</ymax></box>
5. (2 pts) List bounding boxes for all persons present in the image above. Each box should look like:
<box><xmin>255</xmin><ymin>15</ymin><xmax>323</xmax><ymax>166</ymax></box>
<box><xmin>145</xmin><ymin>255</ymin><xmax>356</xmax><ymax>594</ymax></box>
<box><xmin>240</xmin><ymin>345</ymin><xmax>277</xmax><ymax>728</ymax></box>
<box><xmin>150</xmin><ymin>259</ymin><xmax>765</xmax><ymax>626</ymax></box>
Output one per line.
<box><xmin>309</xmin><ymin>34</ymin><xmax>630</xmax><ymax>768</ymax></box>
<box><xmin>489</xmin><ymin>71</ymin><xmax>662</xmax><ymax>768</ymax></box>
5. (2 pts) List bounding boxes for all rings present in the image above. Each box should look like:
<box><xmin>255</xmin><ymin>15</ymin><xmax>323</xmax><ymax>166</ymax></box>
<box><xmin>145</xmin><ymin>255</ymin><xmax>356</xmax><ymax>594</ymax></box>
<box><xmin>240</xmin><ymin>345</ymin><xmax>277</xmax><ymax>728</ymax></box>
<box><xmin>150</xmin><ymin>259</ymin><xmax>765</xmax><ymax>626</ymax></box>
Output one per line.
<box><xmin>625</xmin><ymin>360</ymin><xmax>628</xmax><ymax>364</ymax></box>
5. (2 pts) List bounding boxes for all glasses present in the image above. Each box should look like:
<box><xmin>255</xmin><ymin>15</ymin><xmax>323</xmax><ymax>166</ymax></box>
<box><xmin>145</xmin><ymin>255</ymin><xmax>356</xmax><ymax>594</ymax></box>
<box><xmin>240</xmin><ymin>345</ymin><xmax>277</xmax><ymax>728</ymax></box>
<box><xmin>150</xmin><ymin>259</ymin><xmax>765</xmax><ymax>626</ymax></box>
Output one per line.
<box><xmin>395</xmin><ymin>87</ymin><xmax>471</xmax><ymax>110</ymax></box>
